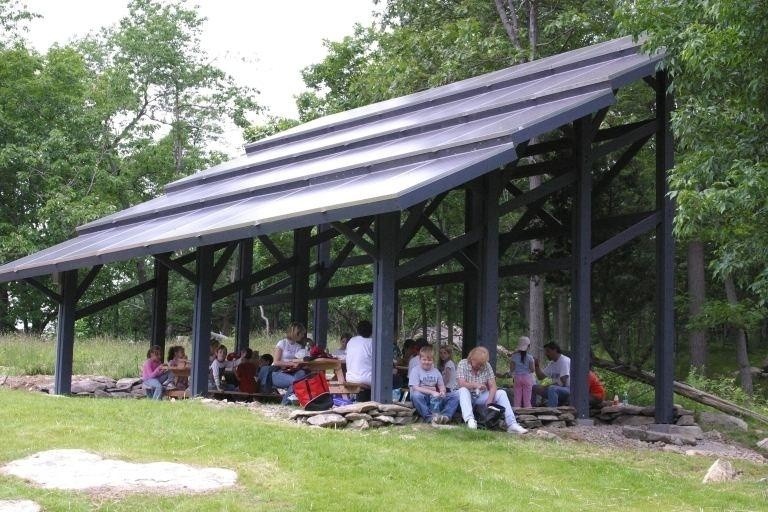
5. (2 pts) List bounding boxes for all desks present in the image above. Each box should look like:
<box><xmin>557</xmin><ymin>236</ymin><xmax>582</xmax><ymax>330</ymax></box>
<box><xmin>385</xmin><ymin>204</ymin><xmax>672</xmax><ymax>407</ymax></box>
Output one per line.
<box><xmin>162</xmin><ymin>366</ymin><xmax>237</xmax><ymax>385</ymax></box>
<box><xmin>283</xmin><ymin>356</ymin><xmax>407</xmax><ymax>403</ymax></box>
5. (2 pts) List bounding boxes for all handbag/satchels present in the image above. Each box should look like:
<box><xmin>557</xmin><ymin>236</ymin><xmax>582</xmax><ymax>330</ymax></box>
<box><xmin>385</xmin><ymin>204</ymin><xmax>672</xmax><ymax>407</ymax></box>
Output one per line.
<box><xmin>293</xmin><ymin>370</ymin><xmax>333</xmax><ymax>411</ymax></box>
<box><xmin>473</xmin><ymin>403</ymin><xmax>505</xmax><ymax>429</ymax></box>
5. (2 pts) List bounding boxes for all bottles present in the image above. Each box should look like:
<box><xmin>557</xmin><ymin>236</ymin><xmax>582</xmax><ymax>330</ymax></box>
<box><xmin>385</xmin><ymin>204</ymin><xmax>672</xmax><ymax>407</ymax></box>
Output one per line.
<box><xmin>622</xmin><ymin>391</ymin><xmax>628</xmax><ymax>405</ymax></box>
<box><xmin>304</xmin><ymin>342</ymin><xmax>310</xmax><ymax>358</ymax></box>
<box><xmin>232</xmin><ymin>358</ymin><xmax>237</xmax><ymax>370</ymax></box>
<box><xmin>393</xmin><ymin>350</ymin><xmax>404</xmax><ymax>366</ymax></box>
<box><xmin>323</xmin><ymin>347</ymin><xmax>329</xmax><ymax>355</ymax></box>
<box><xmin>613</xmin><ymin>394</ymin><xmax>618</xmax><ymax>405</ymax></box>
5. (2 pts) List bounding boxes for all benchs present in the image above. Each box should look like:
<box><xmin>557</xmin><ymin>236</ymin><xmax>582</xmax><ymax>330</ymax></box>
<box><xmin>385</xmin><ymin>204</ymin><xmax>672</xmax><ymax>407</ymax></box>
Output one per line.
<box><xmin>144</xmin><ymin>381</ymin><xmax>410</xmax><ymax>406</ymax></box>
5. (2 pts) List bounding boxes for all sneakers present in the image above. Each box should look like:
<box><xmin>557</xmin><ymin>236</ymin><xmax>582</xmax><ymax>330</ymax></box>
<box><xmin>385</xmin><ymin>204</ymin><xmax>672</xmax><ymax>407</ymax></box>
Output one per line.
<box><xmin>507</xmin><ymin>423</ymin><xmax>528</xmax><ymax>434</ymax></box>
<box><xmin>467</xmin><ymin>419</ymin><xmax>477</xmax><ymax>429</ymax></box>
<box><xmin>443</xmin><ymin>416</ymin><xmax>449</xmax><ymax>424</ymax></box>
<box><xmin>431</xmin><ymin>414</ymin><xmax>443</xmax><ymax>424</ymax></box>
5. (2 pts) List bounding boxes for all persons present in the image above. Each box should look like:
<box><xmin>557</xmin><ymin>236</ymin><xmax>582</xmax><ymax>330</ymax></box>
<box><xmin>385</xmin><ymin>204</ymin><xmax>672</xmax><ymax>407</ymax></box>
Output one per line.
<box><xmin>209</xmin><ymin>339</ymin><xmax>274</xmax><ymax>392</ymax></box>
<box><xmin>589</xmin><ymin>370</ymin><xmax>604</xmax><ymax>406</ymax></box>
<box><xmin>393</xmin><ymin>338</ymin><xmax>460</xmax><ymax>425</ymax></box>
<box><xmin>334</xmin><ymin>320</ymin><xmax>372</xmax><ymax>386</ymax></box>
<box><xmin>531</xmin><ymin>343</ymin><xmax>571</xmax><ymax>408</ymax></box>
<box><xmin>510</xmin><ymin>336</ymin><xmax>535</xmax><ymax>408</ymax></box>
<box><xmin>453</xmin><ymin>346</ymin><xmax>527</xmax><ymax>434</ymax></box>
<box><xmin>272</xmin><ymin>321</ymin><xmax>307</xmax><ymax>403</ymax></box>
<box><xmin>143</xmin><ymin>345</ymin><xmax>188</xmax><ymax>400</ymax></box>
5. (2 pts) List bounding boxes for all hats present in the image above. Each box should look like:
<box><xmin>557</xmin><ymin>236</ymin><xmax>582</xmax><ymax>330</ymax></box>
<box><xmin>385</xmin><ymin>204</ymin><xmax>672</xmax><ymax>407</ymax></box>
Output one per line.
<box><xmin>515</xmin><ymin>336</ymin><xmax>531</xmax><ymax>351</ymax></box>
